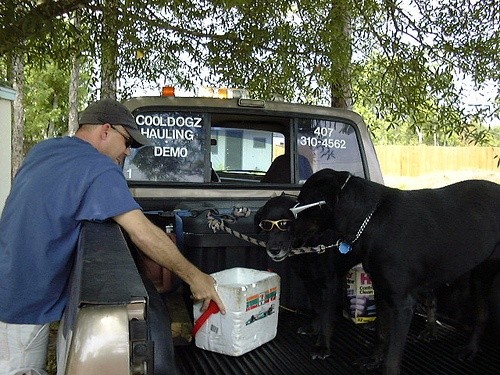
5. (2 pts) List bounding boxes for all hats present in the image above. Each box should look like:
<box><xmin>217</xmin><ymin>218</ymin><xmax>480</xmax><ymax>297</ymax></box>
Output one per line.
<box><xmin>78</xmin><ymin>98</ymin><xmax>149</xmax><ymax>149</ymax></box>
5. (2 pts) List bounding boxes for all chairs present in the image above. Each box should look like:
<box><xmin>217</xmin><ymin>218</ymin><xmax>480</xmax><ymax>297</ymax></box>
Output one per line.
<box><xmin>262</xmin><ymin>153</ymin><xmax>313</xmax><ymax>181</ymax></box>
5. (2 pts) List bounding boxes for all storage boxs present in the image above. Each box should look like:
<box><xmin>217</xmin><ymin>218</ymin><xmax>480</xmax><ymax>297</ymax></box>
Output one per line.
<box><xmin>193</xmin><ymin>263</ymin><xmax>281</xmax><ymax>356</ymax></box>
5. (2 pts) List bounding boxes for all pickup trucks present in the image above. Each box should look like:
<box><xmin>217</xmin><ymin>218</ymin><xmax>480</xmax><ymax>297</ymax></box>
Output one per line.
<box><xmin>53</xmin><ymin>82</ymin><xmax>500</xmax><ymax>375</ymax></box>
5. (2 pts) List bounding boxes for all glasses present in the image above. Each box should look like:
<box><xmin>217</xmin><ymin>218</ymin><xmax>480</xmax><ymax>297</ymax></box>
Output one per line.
<box><xmin>259</xmin><ymin>220</ymin><xmax>291</xmax><ymax>232</ymax></box>
<box><xmin>290</xmin><ymin>200</ymin><xmax>326</xmax><ymax>219</ymax></box>
<box><xmin>96</xmin><ymin>116</ymin><xmax>136</xmax><ymax>148</ymax></box>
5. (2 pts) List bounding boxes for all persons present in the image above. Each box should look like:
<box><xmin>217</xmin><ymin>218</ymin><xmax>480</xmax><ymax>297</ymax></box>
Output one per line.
<box><xmin>0</xmin><ymin>98</ymin><xmax>226</xmax><ymax>375</ymax></box>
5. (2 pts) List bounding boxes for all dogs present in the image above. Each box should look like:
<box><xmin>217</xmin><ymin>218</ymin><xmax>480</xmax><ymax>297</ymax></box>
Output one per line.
<box><xmin>253</xmin><ymin>193</ymin><xmax>438</xmax><ymax>359</ymax></box>
<box><xmin>298</xmin><ymin>168</ymin><xmax>500</xmax><ymax>374</ymax></box>
